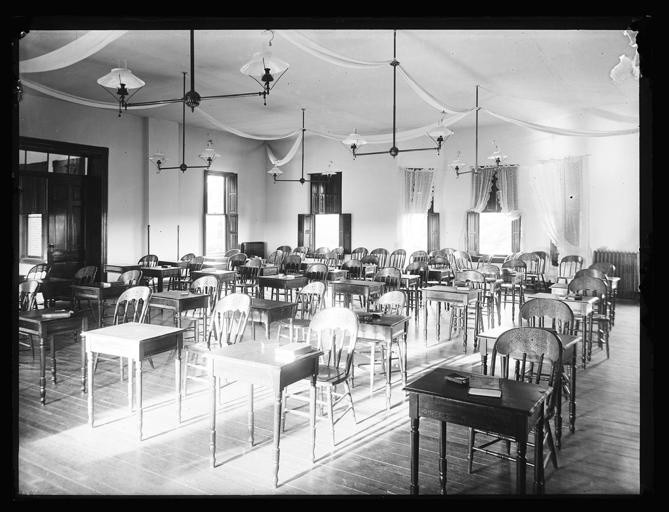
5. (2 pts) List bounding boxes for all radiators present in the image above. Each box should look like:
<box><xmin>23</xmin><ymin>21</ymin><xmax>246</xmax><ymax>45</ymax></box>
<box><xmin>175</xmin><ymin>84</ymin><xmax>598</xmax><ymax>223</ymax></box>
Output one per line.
<box><xmin>593</xmin><ymin>248</ymin><xmax>637</xmax><ymax>299</ymax></box>
<box><xmin>241</xmin><ymin>242</ymin><xmax>264</xmax><ymax>259</ymax></box>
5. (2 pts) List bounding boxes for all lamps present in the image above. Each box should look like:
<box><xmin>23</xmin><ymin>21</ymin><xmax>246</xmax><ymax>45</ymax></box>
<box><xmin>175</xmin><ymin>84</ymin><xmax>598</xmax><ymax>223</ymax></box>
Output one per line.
<box><xmin>266</xmin><ymin>108</ymin><xmax>341</xmax><ymax>185</ymax></box>
<box><xmin>343</xmin><ymin>29</ymin><xmax>454</xmax><ymax>160</ymax></box>
<box><xmin>97</xmin><ymin>30</ymin><xmax>289</xmax><ymax>119</ymax></box>
<box><xmin>450</xmin><ymin>86</ymin><xmax>506</xmax><ymax>177</ymax></box>
<box><xmin>149</xmin><ymin>71</ymin><xmax>221</xmax><ymax>174</ymax></box>
